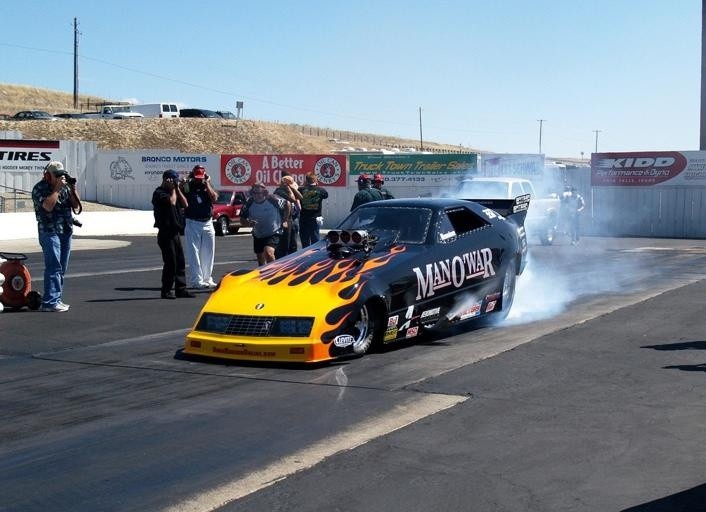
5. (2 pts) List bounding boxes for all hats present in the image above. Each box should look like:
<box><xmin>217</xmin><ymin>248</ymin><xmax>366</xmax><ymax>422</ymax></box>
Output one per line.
<box><xmin>192</xmin><ymin>168</ymin><xmax>206</xmax><ymax>179</ymax></box>
<box><xmin>163</xmin><ymin>170</ymin><xmax>183</xmax><ymax>182</ymax></box>
<box><xmin>281</xmin><ymin>176</ymin><xmax>299</xmax><ymax>190</ymax></box>
<box><xmin>47</xmin><ymin>161</ymin><xmax>68</xmax><ymax>175</ymax></box>
<box><xmin>355</xmin><ymin>174</ymin><xmax>371</xmax><ymax>182</ymax></box>
<box><xmin>375</xmin><ymin>174</ymin><xmax>384</xmax><ymax>181</ymax></box>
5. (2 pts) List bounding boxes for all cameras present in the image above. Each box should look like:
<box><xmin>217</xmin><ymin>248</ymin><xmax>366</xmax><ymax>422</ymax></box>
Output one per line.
<box><xmin>63</xmin><ymin>174</ymin><xmax>76</xmax><ymax>184</ymax></box>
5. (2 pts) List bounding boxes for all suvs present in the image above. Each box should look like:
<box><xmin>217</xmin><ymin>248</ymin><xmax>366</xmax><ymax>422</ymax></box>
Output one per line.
<box><xmin>176</xmin><ymin>188</ymin><xmax>254</xmax><ymax>236</ymax></box>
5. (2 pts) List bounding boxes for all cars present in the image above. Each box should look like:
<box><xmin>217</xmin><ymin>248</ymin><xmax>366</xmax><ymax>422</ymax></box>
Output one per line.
<box><xmin>0</xmin><ymin>103</ymin><xmax>239</xmax><ymax>123</ymax></box>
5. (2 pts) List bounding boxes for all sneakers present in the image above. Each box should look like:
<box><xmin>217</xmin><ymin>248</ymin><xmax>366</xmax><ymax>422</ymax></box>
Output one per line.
<box><xmin>166</xmin><ymin>293</ymin><xmax>176</xmax><ymax>299</ymax></box>
<box><xmin>42</xmin><ymin>301</ymin><xmax>71</xmax><ymax>312</ymax></box>
<box><xmin>193</xmin><ymin>282</ymin><xmax>218</xmax><ymax>289</ymax></box>
<box><xmin>175</xmin><ymin>293</ymin><xmax>195</xmax><ymax>298</ymax></box>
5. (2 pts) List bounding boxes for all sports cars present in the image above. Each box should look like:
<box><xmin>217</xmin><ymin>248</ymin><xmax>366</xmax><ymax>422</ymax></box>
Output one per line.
<box><xmin>183</xmin><ymin>193</ymin><xmax>542</xmax><ymax>368</ymax></box>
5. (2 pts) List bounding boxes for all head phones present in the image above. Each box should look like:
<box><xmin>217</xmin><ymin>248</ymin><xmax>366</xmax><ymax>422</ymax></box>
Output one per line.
<box><xmin>358</xmin><ymin>178</ymin><xmax>363</xmax><ymax>185</ymax></box>
<box><xmin>371</xmin><ymin>174</ymin><xmax>384</xmax><ymax>184</ymax></box>
<box><xmin>189</xmin><ymin>165</ymin><xmax>208</xmax><ymax>179</ymax></box>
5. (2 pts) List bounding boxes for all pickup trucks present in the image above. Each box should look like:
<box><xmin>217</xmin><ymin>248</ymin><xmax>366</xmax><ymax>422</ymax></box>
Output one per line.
<box><xmin>446</xmin><ymin>175</ymin><xmax>568</xmax><ymax>248</ymax></box>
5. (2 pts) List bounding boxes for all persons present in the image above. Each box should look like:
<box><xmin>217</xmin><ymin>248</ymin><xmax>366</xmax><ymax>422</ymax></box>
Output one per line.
<box><xmin>565</xmin><ymin>188</ymin><xmax>584</xmax><ymax>244</ymax></box>
<box><xmin>239</xmin><ymin>169</ymin><xmax>394</xmax><ymax>268</ymax></box>
<box><xmin>32</xmin><ymin>161</ymin><xmax>80</xmax><ymax>312</ymax></box>
<box><xmin>180</xmin><ymin>165</ymin><xmax>219</xmax><ymax>289</ymax></box>
<box><xmin>152</xmin><ymin>169</ymin><xmax>196</xmax><ymax>299</ymax></box>
